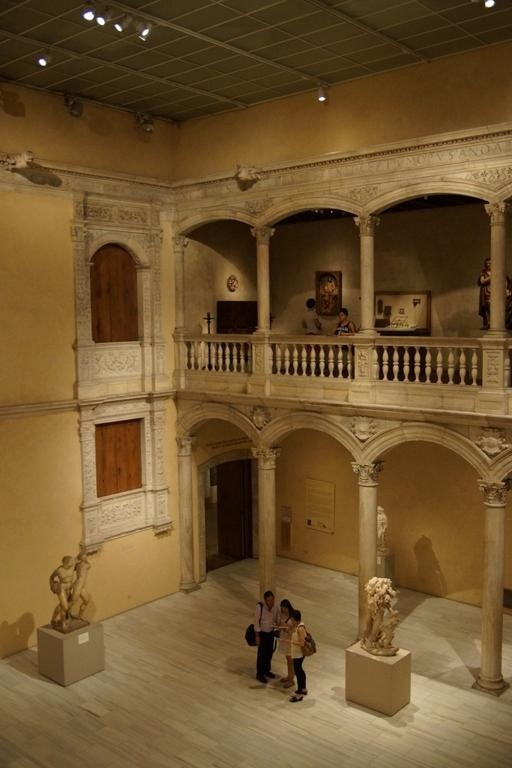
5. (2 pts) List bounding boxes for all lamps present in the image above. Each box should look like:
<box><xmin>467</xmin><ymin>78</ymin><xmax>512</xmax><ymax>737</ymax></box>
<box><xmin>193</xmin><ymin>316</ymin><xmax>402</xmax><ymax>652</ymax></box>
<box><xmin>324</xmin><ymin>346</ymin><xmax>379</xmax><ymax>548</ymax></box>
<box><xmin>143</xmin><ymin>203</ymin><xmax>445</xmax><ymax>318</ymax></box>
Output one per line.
<box><xmin>39</xmin><ymin>51</ymin><xmax>51</xmax><ymax>67</ymax></box>
<box><xmin>63</xmin><ymin>94</ymin><xmax>83</xmax><ymax>118</ymax></box>
<box><xmin>316</xmin><ymin>83</ymin><xmax>328</xmax><ymax>102</ymax></box>
<box><xmin>137</xmin><ymin>111</ymin><xmax>155</xmax><ymax>133</ymax></box>
<box><xmin>82</xmin><ymin>1</ymin><xmax>153</xmax><ymax>42</ymax></box>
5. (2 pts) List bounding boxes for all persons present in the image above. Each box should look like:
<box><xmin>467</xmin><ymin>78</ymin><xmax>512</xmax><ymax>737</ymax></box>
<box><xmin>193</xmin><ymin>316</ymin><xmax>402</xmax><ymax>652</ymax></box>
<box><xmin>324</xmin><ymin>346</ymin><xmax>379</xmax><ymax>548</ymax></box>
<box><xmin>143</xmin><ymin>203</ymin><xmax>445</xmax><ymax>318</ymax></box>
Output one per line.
<box><xmin>301</xmin><ymin>297</ymin><xmax>324</xmax><ymax>372</ymax></box>
<box><xmin>65</xmin><ymin>550</ymin><xmax>92</xmax><ymax>623</ymax></box>
<box><xmin>332</xmin><ymin>306</ymin><xmax>357</xmax><ymax>375</ymax></box>
<box><xmin>48</xmin><ymin>556</ymin><xmax>80</xmax><ymax>630</ymax></box>
<box><xmin>323</xmin><ymin>276</ymin><xmax>338</xmax><ymax>310</ymax></box>
<box><xmin>269</xmin><ymin>598</ymin><xmax>295</xmax><ymax>689</ymax></box>
<box><xmin>478</xmin><ymin>258</ymin><xmax>491</xmax><ymax>331</ymax></box>
<box><xmin>288</xmin><ymin>610</ymin><xmax>308</xmax><ymax>703</ymax></box>
<box><xmin>377</xmin><ymin>504</ymin><xmax>389</xmax><ymax>553</ymax></box>
<box><xmin>253</xmin><ymin>590</ymin><xmax>280</xmax><ymax>684</ymax></box>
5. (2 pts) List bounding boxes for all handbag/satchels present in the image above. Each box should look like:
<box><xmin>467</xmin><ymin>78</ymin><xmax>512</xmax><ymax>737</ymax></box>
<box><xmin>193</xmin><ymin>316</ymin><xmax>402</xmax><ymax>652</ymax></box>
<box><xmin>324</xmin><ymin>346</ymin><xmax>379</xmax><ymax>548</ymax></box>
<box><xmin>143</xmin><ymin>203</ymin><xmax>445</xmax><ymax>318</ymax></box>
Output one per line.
<box><xmin>302</xmin><ymin>633</ymin><xmax>316</xmax><ymax>656</ymax></box>
<box><xmin>274</xmin><ymin>630</ymin><xmax>280</xmax><ymax>638</ymax></box>
<box><xmin>246</xmin><ymin>624</ymin><xmax>256</xmax><ymax>645</ymax></box>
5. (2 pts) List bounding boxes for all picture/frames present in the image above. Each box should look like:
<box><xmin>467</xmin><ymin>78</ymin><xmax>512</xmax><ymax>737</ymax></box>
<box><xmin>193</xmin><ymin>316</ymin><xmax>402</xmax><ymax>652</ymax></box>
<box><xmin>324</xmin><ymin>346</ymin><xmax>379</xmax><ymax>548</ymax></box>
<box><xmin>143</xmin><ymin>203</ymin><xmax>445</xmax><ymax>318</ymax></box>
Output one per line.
<box><xmin>374</xmin><ymin>290</ymin><xmax>431</xmax><ymax>334</ymax></box>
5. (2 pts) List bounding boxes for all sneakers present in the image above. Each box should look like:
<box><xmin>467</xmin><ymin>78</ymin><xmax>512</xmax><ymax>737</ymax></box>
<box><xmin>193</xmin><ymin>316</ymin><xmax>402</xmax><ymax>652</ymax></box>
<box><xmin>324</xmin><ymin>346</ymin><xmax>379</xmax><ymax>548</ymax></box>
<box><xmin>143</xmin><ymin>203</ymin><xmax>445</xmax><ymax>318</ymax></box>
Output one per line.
<box><xmin>256</xmin><ymin>671</ymin><xmax>275</xmax><ymax>683</ymax></box>
<box><xmin>280</xmin><ymin>677</ymin><xmax>294</xmax><ymax>687</ymax></box>
<box><xmin>290</xmin><ymin>690</ymin><xmax>307</xmax><ymax>702</ymax></box>
